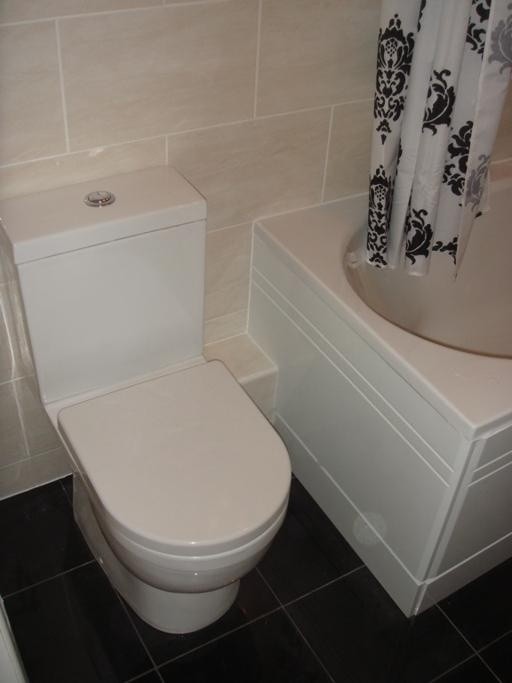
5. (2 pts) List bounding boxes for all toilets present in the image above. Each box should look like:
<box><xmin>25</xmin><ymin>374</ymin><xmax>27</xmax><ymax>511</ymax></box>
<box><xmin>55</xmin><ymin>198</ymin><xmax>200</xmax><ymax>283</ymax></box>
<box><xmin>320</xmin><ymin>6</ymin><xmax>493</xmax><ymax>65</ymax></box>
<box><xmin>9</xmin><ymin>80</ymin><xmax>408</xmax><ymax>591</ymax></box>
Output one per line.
<box><xmin>0</xmin><ymin>163</ymin><xmax>295</xmax><ymax>638</ymax></box>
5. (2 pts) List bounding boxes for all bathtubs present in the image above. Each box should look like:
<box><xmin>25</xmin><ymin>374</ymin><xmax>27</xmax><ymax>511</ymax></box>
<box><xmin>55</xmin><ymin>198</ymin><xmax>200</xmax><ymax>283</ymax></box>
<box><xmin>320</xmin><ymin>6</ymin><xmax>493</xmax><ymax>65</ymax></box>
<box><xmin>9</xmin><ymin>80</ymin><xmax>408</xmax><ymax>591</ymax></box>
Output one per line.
<box><xmin>247</xmin><ymin>161</ymin><xmax>512</xmax><ymax>621</ymax></box>
